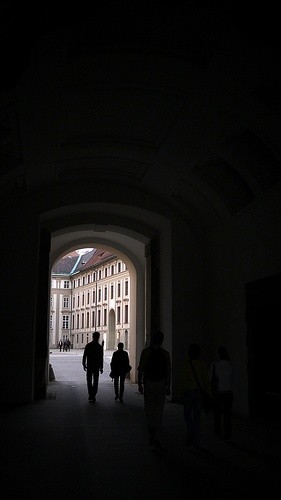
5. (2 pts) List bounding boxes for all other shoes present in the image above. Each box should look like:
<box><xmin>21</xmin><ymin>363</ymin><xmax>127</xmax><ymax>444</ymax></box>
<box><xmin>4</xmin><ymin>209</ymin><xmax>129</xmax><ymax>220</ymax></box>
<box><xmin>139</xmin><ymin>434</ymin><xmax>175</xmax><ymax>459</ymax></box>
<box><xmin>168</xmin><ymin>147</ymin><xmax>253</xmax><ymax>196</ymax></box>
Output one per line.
<box><xmin>89</xmin><ymin>395</ymin><xmax>96</xmax><ymax>401</ymax></box>
<box><xmin>119</xmin><ymin>396</ymin><xmax>123</xmax><ymax>399</ymax></box>
<box><xmin>195</xmin><ymin>438</ymin><xmax>201</xmax><ymax>444</ymax></box>
<box><xmin>184</xmin><ymin>440</ymin><xmax>193</xmax><ymax>449</ymax></box>
<box><xmin>223</xmin><ymin>437</ymin><xmax>231</xmax><ymax>442</ymax></box>
<box><xmin>115</xmin><ymin>394</ymin><xmax>119</xmax><ymax>400</ymax></box>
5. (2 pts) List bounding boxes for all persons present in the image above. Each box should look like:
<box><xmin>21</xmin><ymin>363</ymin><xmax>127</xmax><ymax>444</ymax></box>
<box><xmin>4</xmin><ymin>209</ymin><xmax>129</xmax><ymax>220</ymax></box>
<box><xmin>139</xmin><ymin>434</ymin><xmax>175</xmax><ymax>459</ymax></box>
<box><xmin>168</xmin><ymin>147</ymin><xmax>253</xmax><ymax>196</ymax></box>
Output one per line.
<box><xmin>110</xmin><ymin>343</ymin><xmax>129</xmax><ymax>402</ymax></box>
<box><xmin>83</xmin><ymin>332</ymin><xmax>103</xmax><ymax>403</ymax></box>
<box><xmin>179</xmin><ymin>343</ymin><xmax>208</xmax><ymax>448</ymax></box>
<box><xmin>209</xmin><ymin>344</ymin><xmax>235</xmax><ymax>444</ymax></box>
<box><xmin>58</xmin><ymin>339</ymin><xmax>63</xmax><ymax>352</ymax></box>
<box><xmin>137</xmin><ymin>331</ymin><xmax>172</xmax><ymax>435</ymax></box>
<box><xmin>63</xmin><ymin>339</ymin><xmax>71</xmax><ymax>352</ymax></box>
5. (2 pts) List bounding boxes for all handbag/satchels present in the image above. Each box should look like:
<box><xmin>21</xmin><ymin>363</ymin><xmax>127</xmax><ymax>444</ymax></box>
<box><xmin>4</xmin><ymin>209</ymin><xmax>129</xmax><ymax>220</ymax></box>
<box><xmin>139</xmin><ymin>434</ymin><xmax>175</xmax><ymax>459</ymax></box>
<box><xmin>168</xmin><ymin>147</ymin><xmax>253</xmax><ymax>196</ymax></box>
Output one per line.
<box><xmin>202</xmin><ymin>390</ymin><xmax>213</xmax><ymax>415</ymax></box>
<box><xmin>211</xmin><ymin>363</ymin><xmax>219</xmax><ymax>395</ymax></box>
